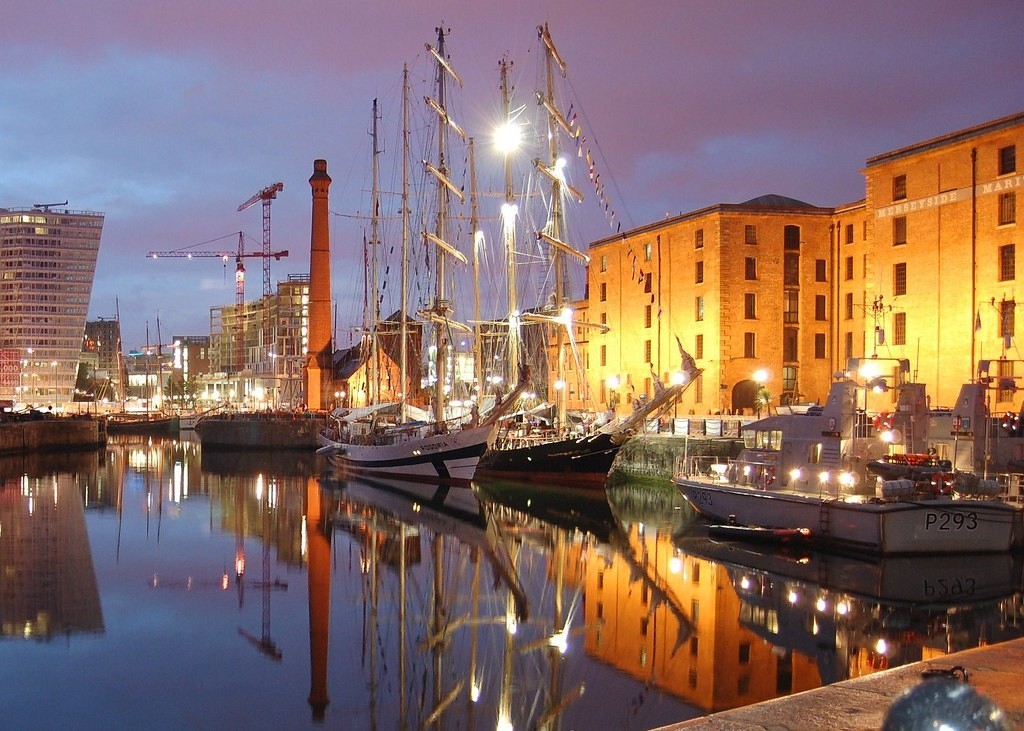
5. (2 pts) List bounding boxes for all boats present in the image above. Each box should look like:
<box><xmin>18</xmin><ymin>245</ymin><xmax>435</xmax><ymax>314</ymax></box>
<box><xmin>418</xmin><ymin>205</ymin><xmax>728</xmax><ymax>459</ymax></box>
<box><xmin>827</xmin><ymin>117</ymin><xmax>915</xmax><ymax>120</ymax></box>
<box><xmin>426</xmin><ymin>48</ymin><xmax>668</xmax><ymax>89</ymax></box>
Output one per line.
<box><xmin>107</xmin><ymin>312</ymin><xmax>182</xmax><ymax>434</ymax></box>
<box><xmin>668</xmin><ymin>518</ymin><xmax>1018</xmax><ymax>699</ymax></box>
<box><xmin>864</xmin><ymin>453</ymin><xmax>954</xmax><ymax>483</ymax></box>
<box><xmin>672</xmin><ymin>293</ymin><xmax>1019</xmax><ymax>558</ymax></box>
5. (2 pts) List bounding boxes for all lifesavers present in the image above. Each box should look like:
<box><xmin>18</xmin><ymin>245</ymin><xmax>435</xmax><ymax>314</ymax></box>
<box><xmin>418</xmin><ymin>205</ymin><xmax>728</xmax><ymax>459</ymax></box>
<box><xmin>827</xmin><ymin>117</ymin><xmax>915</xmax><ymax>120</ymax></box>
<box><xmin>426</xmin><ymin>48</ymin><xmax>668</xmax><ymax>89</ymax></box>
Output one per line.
<box><xmin>875</xmin><ymin>412</ymin><xmax>895</xmax><ymax>433</ymax></box>
<box><xmin>930</xmin><ymin>473</ymin><xmax>955</xmax><ymax>494</ymax></box>
<box><xmin>761</xmin><ymin>466</ymin><xmax>777</xmax><ymax>485</ymax></box>
<box><xmin>1000</xmin><ymin>413</ymin><xmax>1019</xmax><ymax>432</ymax></box>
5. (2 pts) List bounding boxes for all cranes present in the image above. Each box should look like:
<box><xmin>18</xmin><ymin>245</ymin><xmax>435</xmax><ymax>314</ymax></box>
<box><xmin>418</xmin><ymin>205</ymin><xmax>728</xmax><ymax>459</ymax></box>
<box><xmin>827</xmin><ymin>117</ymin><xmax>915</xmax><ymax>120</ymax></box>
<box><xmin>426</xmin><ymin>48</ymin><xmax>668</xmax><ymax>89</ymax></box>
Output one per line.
<box><xmin>235</xmin><ymin>181</ymin><xmax>285</xmax><ymax>374</ymax></box>
<box><xmin>147</xmin><ymin>229</ymin><xmax>288</xmax><ymax>373</ymax></box>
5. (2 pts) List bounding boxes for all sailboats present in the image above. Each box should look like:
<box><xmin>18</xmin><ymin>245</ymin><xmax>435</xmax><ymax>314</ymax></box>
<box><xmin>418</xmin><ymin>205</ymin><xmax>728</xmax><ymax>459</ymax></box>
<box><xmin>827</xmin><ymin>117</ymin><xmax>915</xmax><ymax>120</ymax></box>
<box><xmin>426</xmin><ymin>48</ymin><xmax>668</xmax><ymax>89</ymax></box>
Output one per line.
<box><xmin>303</xmin><ymin>462</ymin><xmax>697</xmax><ymax>730</ymax></box>
<box><xmin>313</xmin><ymin>16</ymin><xmax>707</xmax><ymax>490</ymax></box>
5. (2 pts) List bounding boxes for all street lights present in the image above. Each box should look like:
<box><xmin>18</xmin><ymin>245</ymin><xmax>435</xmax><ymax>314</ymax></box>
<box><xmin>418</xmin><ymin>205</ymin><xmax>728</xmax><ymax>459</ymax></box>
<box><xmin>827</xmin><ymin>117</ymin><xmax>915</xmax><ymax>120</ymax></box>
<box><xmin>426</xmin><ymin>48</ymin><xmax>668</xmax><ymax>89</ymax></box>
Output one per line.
<box><xmin>52</xmin><ymin>361</ymin><xmax>58</xmax><ymax>419</ymax></box>
